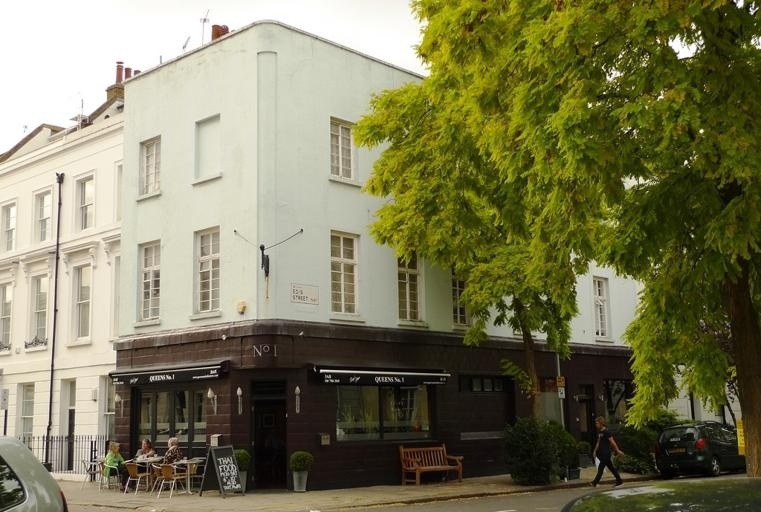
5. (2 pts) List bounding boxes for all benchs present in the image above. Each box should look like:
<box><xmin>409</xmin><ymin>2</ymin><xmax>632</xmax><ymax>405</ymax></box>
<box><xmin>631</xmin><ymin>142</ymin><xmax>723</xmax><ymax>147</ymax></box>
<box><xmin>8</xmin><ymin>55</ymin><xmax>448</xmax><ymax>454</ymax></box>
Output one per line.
<box><xmin>398</xmin><ymin>443</ymin><xmax>465</xmax><ymax>486</ymax></box>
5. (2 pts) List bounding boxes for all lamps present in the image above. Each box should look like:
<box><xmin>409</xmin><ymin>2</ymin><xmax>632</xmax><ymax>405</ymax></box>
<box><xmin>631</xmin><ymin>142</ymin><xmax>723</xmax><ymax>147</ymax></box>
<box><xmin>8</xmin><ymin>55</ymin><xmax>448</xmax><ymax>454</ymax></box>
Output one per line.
<box><xmin>114</xmin><ymin>393</ymin><xmax>124</xmax><ymax>418</ymax></box>
<box><xmin>208</xmin><ymin>386</ymin><xmax>300</xmax><ymax>415</ymax></box>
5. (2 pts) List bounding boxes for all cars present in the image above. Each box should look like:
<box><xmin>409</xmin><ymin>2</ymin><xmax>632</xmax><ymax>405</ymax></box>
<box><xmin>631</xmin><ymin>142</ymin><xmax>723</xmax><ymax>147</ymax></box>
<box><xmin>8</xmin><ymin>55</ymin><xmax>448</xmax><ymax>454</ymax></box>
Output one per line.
<box><xmin>1</xmin><ymin>435</ymin><xmax>69</xmax><ymax>511</ymax></box>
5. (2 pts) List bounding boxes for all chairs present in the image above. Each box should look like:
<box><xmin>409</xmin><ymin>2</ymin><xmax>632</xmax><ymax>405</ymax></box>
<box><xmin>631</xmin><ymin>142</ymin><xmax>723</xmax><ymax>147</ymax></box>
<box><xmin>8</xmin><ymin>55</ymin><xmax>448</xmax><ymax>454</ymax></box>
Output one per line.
<box><xmin>81</xmin><ymin>456</ymin><xmax>206</xmax><ymax>499</ymax></box>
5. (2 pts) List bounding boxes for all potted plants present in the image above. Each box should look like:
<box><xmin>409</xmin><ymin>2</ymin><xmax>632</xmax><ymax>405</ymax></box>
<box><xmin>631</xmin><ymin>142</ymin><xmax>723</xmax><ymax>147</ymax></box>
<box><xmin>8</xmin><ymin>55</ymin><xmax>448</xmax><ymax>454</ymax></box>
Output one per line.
<box><xmin>548</xmin><ymin>421</ymin><xmax>590</xmax><ymax>483</ymax></box>
<box><xmin>231</xmin><ymin>449</ymin><xmax>251</xmax><ymax>494</ymax></box>
<box><xmin>288</xmin><ymin>451</ymin><xmax>314</xmax><ymax>492</ymax></box>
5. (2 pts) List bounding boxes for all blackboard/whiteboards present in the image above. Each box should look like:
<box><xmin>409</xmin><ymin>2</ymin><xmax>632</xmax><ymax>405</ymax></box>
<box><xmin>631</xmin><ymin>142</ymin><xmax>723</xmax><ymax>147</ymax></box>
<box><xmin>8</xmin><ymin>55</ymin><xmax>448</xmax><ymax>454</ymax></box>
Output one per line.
<box><xmin>211</xmin><ymin>446</ymin><xmax>243</xmax><ymax>493</ymax></box>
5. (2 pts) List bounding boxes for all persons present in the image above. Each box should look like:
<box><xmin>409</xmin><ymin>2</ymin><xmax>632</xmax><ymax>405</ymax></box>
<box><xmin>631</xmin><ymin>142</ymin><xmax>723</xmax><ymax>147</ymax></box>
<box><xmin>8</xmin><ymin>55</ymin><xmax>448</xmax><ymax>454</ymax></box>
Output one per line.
<box><xmin>135</xmin><ymin>439</ymin><xmax>158</xmax><ymax>475</ymax></box>
<box><xmin>102</xmin><ymin>441</ymin><xmax>135</xmax><ymax>492</ymax></box>
<box><xmin>587</xmin><ymin>416</ymin><xmax>625</xmax><ymax>488</ymax></box>
<box><xmin>164</xmin><ymin>437</ymin><xmax>186</xmax><ymax>473</ymax></box>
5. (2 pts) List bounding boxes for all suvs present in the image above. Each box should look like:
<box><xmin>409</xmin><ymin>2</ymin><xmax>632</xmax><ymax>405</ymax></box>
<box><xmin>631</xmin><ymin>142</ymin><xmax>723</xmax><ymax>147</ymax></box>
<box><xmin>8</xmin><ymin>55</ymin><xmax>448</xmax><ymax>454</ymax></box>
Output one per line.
<box><xmin>654</xmin><ymin>420</ymin><xmax>747</xmax><ymax>476</ymax></box>
